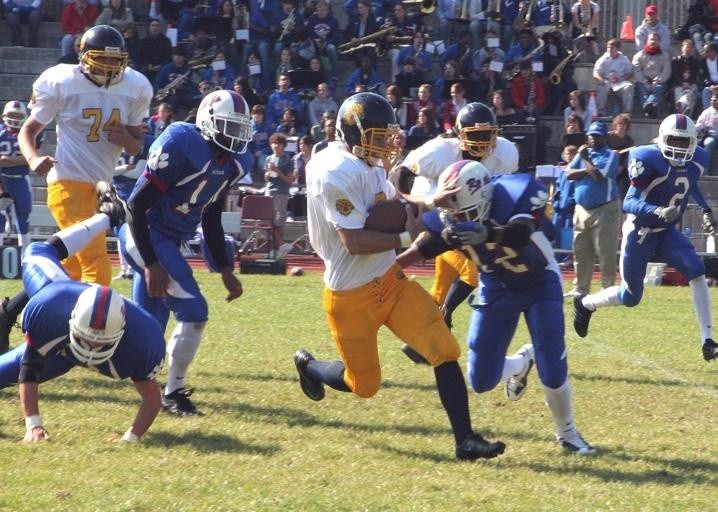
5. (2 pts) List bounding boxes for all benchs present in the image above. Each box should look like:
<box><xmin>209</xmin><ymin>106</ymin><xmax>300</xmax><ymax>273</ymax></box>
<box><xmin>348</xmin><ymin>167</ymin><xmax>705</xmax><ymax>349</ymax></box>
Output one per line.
<box><xmin>186</xmin><ymin>209</ymin><xmax>241</xmax><ymax>259</ymax></box>
<box><xmin>1</xmin><ymin>203</ymin><xmax>117</xmax><ymax>245</ymax></box>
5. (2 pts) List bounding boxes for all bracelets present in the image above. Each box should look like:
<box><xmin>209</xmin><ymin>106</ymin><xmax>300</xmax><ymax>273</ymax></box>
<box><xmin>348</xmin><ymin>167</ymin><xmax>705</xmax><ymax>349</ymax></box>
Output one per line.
<box><xmin>120</xmin><ymin>430</ymin><xmax>137</xmax><ymax>443</ymax></box>
<box><xmin>25</xmin><ymin>414</ymin><xmax>43</xmax><ymax>429</ymax></box>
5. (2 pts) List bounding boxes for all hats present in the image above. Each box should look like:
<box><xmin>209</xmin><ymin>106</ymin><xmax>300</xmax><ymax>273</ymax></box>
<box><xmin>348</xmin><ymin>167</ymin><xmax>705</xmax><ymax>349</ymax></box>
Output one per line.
<box><xmin>644</xmin><ymin>5</ymin><xmax>657</xmax><ymax>15</ymax></box>
<box><xmin>586</xmin><ymin>121</ymin><xmax>608</xmax><ymax>138</ymax></box>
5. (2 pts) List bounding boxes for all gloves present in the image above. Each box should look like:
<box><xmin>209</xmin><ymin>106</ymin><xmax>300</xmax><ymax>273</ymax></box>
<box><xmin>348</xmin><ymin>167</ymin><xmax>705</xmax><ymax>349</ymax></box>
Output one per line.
<box><xmin>453</xmin><ymin>221</ymin><xmax>488</xmax><ymax>245</ymax></box>
<box><xmin>701</xmin><ymin>213</ymin><xmax>716</xmax><ymax>235</ymax></box>
<box><xmin>654</xmin><ymin>205</ymin><xmax>680</xmax><ymax>224</ymax></box>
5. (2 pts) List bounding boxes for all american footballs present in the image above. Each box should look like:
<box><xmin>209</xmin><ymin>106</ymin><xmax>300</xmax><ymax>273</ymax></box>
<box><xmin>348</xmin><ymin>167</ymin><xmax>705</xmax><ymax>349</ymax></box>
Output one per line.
<box><xmin>362</xmin><ymin>198</ymin><xmax>421</xmax><ymax>231</ymax></box>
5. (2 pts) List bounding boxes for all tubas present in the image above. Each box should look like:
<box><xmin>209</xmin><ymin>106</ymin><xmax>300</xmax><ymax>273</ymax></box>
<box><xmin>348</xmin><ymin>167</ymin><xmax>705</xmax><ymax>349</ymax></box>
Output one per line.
<box><xmin>445</xmin><ymin>1</ymin><xmax>471</xmax><ymax>47</ymax></box>
<box><xmin>402</xmin><ymin>0</ymin><xmax>438</xmax><ymax>14</ymax></box>
<box><xmin>511</xmin><ymin>1</ymin><xmax>537</xmax><ymax>48</ymax></box>
<box><xmin>337</xmin><ymin>26</ymin><xmax>398</xmax><ymax>57</ymax></box>
<box><xmin>485</xmin><ymin>1</ymin><xmax>502</xmax><ymax>36</ymax></box>
<box><xmin>504</xmin><ymin>37</ymin><xmax>543</xmax><ymax>80</ymax></box>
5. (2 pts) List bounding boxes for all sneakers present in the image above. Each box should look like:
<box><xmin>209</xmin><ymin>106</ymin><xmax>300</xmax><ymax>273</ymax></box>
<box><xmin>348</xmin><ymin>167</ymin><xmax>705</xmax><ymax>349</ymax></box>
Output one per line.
<box><xmin>564</xmin><ymin>289</ymin><xmax>588</xmax><ymax>298</ymax></box>
<box><xmin>402</xmin><ymin>306</ymin><xmax>450</xmax><ymax>364</ymax></box>
<box><xmin>0</xmin><ymin>296</ymin><xmax>20</xmax><ymax>353</ymax></box>
<box><xmin>293</xmin><ymin>349</ymin><xmax>325</xmax><ymax>401</ymax></box>
<box><xmin>159</xmin><ymin>388</ymin><xmax>204</xmax><ymax>417</ymax></box>
<box><xmin>454</xmin><ymin>433</ymin><xmax>506</xmax><ymax>460</ymax></box>
<box><xmin>556</xmin><ymin>426</ymin><xmax>596</xmax><ymax>454</ymax></box>
<box><xmin>572</xmin><ymin>292</ymin><xmax>594</xmax><ymax>337</ymax></box>
<box><xmin>506</xmin><ymin>344</ymin><xmax>536</xmax><ymax>401</ymax></box>
<box><xmin>94</xmin><ymin>177</ymin><xmax>135</xmax><ymax>231</ymax></box>
<box><xmin>701</xmin><ymin>338</ymin><xmax>718</xmax><ymax>361</ymax></box>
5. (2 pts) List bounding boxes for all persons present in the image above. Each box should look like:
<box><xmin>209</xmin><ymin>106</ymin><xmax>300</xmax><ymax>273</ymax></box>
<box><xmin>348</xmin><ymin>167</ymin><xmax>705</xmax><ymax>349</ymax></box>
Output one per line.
<box><xmin>293</xmin><ymin>91</ymin><xmax>506</xmax><ymax>462</ymax></box>
<box><xmin>562</xmin><ymin>119</ymin><xmax>621</xmax><ymax>300</ymax></box>
<box><xmin>3</xmin><ymin>1</ymin><xmax>42</xmax><ymax>48</ymax></box>
<box><xmin>17</xmin><ymin>23</ymin><xmax>152</xmax><ymax>286</ymax></box>
<box><xmin>0</xmin><ymin>100</ymin><xmax>44</xmax><ymax>261</ymax></box>
<box><xmin>1</xmin><ymin>180</ymin><xmax>167</xmax><ymax>447</ymax></box>
<box><xmin>118</xmin><ymin>90</ymin><xmax>253</xmax><ymax>417</ymax></box>
<box><xmin>392</xmin><ymin>100</ymin><xmax>520</xmax><ymax>363</ymax></box>
<box><xmin>574</xmin><ymin>114</ymin><xmax>718</xmax><ymax>361</ymax></box>
<box><xmin>396</xmin><ymin>157</ymin><xmax>598</xmax><ymax>461</ymax></box>
<box><xmin>59</xmin><ymin>0</ymin><xmax>717</xmax><ymax>262</ymax></box>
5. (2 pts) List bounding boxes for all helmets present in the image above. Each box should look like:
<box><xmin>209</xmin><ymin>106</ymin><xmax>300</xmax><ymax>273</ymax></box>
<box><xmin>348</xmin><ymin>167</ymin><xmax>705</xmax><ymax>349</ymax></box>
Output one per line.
<box><xmin>64</xmin><ymin>285</ymin><xmax>126</xmax><ymax>367</ymax></box>
<box><xmin>78</xmin><ymin>25</ymin><xmax>130</xmax><ymax>85</ymax></box>
<box><xmin>3</xmin><ymin>100</ymin><xmax>29</xmax><ymax>131</ymax></box>
<box><xmin>657</xmin><ymin>113</ymin><xmax>697</xmax><ymax>154</ymax></box>
<box><xmin>196</xmin><ymin>90</ymin><xmax>250</xmax><ymax>141</ymax></box>
<box><xmin>336</xmin><ymin>94</ymin><xmax>499</xmax><ymax>222</ymax></box>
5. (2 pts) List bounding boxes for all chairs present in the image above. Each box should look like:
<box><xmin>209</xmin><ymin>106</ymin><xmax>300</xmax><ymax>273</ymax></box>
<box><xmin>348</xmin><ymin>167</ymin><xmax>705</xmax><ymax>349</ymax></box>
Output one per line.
<box><xmin>284</xmin><ymin>196</ymin><xmax>314</xmax><ymax>256</ymax></box>
<box><xmin>239</xmin><ymin>195</ymin><xmax>278</xmax><ymax>261</ymax></box>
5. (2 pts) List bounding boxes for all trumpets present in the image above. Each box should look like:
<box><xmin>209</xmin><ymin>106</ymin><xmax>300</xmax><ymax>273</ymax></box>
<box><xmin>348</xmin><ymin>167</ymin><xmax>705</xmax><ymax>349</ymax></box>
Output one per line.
<box><xmin>278</xmin><ymin>8</ymin><xmax>296</xmax><ymax>43</ymax></box>
<box><xmin>151</xmin><ymin>68</ymin><xmax>190</xmax><ymax>106</ymax></box>
<box><xmin>550</xmin><ymin>0</ymin><xmax>565</xmax><ymax>28</ymax></box>
<box><xmin>550</xmin><ymin>50</ymin><xmax>572</xmax><ymax>84</ymax></box>
